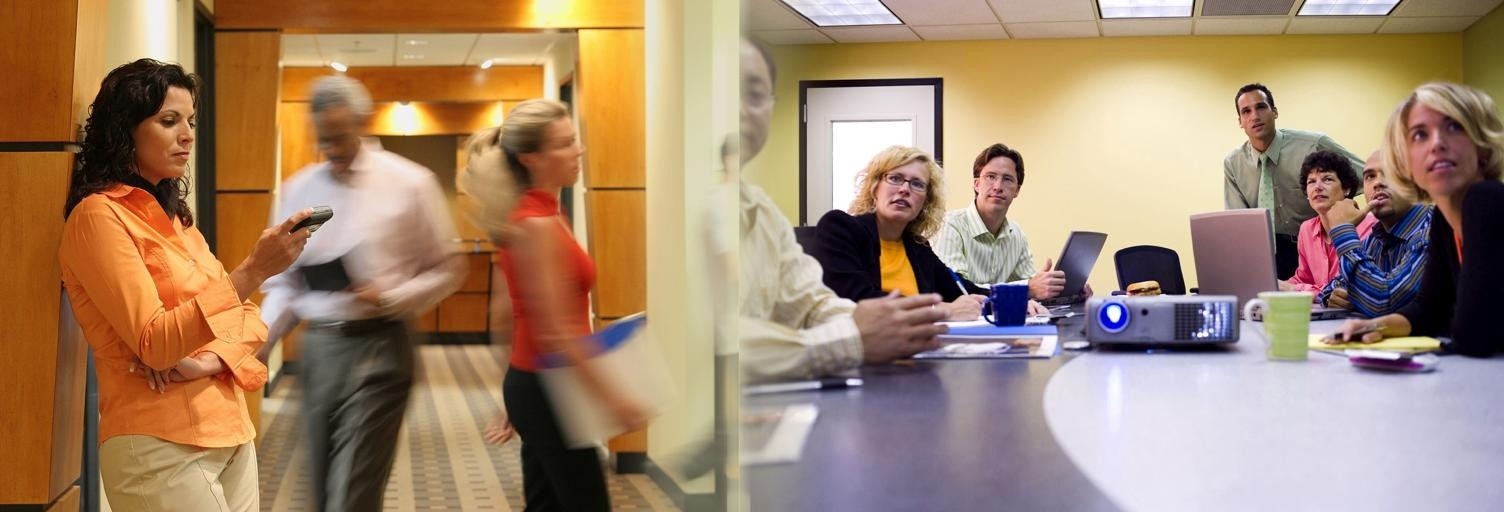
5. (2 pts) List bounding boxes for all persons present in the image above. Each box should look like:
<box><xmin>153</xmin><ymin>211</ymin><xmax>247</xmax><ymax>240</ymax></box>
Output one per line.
<box><xmin>803</xmin><ymin>143</ymin><xmax>1052</xmax><ymax>322</ymax></box>
<box><xmin>927</xmin><ymin>144</ymin><xmax>1096</xmax><ymax>302</ymax></box>
<box><xmin>740</xmin><ymin>28</ymin><xmax>951</xmax><ymax>382</ymax></box>
<box><xmin>1317</xmin><ymin>79</ymin><xmax>1502</xmax><ymax>360</ymax></box>
<box><xmin>1313</xmin><ymin>141</ymin><xmax>1437</xmax><ymax>317</ymax></box>
<box><xmin>252</xmin><ymin>73</ymin><xmax>468</xmax><ymax>511</ymax></box>
<box><xmin>462</xmin><ymin>95</ymin><xmax>653</xmax><ymax>510</ymax></box>
<box><xmin>1276</xmin><ymin>150</ymin><xmax>1382</xmax><ymax>303</ymax></box>
<box><xmin>55</xmin><ymin>56</ymin><xmax>315</xmax><ymax>512</ymax></box>
<box><xmin>1220</xmin><ymin>83</ymin><xmax>1367</xmax><ymax>282</ymax></box>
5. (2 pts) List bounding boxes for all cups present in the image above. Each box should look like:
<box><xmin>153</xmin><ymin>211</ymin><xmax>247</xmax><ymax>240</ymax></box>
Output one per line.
<box><xmin>1244</xmin><ymin>289</ymin><xmax>1314</xmax><ymax>362</ymax></box>
<box><xmin>983</xmin><ymin>282</ymin><xmax>1029</xmax><ymax>327</ymax></box>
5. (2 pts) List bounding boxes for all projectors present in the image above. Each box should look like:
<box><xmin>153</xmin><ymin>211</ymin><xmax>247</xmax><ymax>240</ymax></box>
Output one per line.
<box><xmin>1084</xmin><ymin>293</ymin><xmax>1240</xmax><ymax>348</ymax></box>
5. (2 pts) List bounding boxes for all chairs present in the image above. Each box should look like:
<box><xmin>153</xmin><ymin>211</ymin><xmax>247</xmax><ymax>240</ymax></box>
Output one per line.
<box><xmin>1109</xmin><ymin>245</ymin><xmax>1202</xmax><ymax>298</ymax></box>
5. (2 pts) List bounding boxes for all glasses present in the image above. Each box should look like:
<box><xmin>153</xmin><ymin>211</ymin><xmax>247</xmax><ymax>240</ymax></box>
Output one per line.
<box><xmin>880</xmin><ymin>172</ymin><xmax>930</xmax><ymax>193</ymax></box>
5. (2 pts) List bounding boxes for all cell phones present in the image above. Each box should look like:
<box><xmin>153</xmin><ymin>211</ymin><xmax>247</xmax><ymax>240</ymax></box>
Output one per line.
<box><xmin>290</xmin><ymin>207</ymin><xmax>334</xmax><ymax>236</ymax></box>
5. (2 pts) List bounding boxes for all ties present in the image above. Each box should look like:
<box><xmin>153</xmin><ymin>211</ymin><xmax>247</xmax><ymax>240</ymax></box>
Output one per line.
<box><xmin>1254</xmin><ymin>155</ymin><xmax>1277</xmax><ymax>259</ymax></box>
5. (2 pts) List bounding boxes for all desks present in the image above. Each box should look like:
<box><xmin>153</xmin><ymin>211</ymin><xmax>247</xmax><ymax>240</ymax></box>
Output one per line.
<box><xmin>741</xmin><ymin>299</ymin><xmax>1504</xmax><ymax>512</ymax></box>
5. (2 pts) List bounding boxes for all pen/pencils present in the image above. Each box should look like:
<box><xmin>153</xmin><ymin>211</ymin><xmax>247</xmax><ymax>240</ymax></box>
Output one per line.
<box><xmin>951</xmin><ymin>272</ymin><xmax>968</xmax><ymax>295</ymax></box>
<box><xmin>1318</xmin><ymin>321</ymin><xmax>1391</xmax><ymax>343</ymax></box>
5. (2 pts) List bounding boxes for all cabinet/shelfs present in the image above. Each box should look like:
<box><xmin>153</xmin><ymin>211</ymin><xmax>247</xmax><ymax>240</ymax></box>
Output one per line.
<box><xmin>403</xmin><ymin>248</ymin><xmax>500</xmax><ymax>346</ymax></box>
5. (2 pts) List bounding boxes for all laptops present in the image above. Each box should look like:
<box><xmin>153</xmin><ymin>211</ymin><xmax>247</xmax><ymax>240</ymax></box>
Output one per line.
<box><xmin>1189</xmin><ymin>207</ymin><xmax>1350</xmax><ymax>321</ymax></box>
<box><xmin>1024</xmin><ymin>231</ymin><xmax>1107</xmax><ymax>306</ymax></box>
